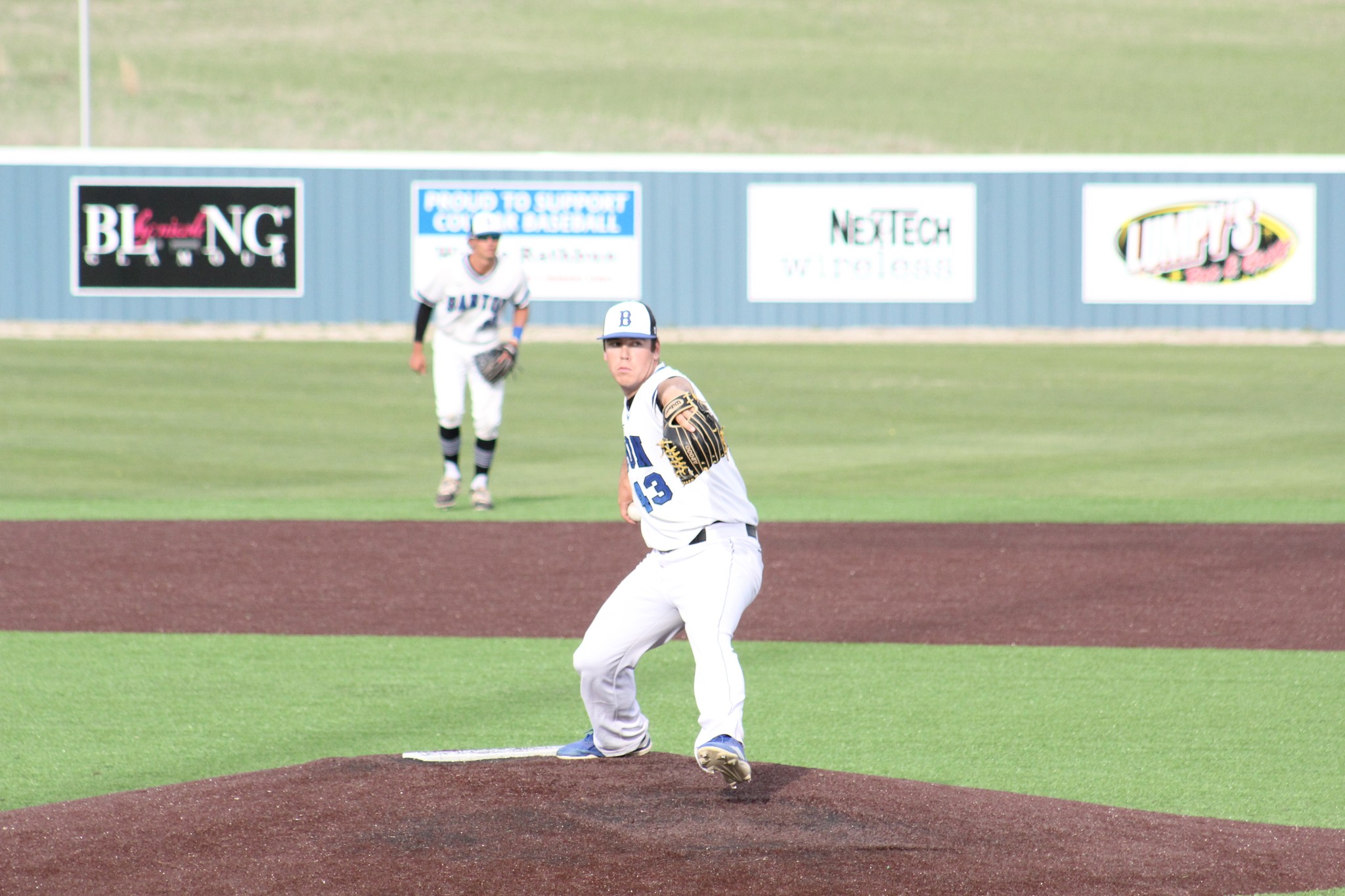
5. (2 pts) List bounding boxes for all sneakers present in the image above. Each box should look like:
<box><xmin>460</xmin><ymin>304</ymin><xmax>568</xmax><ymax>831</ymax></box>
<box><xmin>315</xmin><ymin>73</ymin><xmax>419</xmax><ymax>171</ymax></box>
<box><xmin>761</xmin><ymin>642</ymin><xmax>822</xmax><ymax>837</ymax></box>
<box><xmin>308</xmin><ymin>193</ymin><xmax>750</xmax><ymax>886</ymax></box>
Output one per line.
<box><xmin>555</xmin><ymin>728</ymin><xmax>653</xmax><ymax>760</ymax></box>
<box><xmin>697</xmin><ymin>734</ymin><xmax>752</xmax><ymax>790</ymax></box>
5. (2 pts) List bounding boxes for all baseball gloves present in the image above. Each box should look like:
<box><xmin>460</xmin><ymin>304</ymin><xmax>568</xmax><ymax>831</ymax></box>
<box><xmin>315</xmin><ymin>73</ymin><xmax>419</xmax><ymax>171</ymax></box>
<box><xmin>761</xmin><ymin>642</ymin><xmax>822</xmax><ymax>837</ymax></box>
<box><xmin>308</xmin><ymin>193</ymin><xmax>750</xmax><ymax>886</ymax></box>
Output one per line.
<box><xmin>474</xmin><ymin>342</ymin><xmax>518</xmax><ymax>384</ymax></box>
<box><xmin>656</xmin><ymin>391</ymin><xmax>728</xmax><ymax>486</ymax></box>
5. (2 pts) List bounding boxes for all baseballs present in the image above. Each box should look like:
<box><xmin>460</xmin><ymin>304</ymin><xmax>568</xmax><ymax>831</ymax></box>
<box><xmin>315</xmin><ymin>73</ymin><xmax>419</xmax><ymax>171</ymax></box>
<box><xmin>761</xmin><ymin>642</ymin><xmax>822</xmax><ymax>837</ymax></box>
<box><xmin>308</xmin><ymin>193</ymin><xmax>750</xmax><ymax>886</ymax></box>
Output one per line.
<box><xmin>627</xmin><ymin>502</ymin><xmax>642</xmax><ymax>522</ymax></box>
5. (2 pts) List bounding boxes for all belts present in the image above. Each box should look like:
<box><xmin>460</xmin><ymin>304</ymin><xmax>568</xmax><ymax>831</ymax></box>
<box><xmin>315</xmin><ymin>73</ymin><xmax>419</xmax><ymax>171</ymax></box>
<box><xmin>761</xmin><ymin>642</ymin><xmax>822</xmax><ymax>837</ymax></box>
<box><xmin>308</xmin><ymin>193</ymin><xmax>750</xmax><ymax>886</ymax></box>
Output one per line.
<box><xmin>660</xmin><ymin>524</ymin><xmax>757</xmax><ymax>556</ymax></box>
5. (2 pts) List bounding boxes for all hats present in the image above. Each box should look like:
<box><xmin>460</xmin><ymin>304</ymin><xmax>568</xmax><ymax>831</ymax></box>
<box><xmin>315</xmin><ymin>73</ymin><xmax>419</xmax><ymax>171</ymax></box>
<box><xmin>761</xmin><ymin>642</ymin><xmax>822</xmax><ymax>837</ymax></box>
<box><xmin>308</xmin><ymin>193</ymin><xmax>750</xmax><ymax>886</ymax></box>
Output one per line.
<box><xmin>596</xmin><ymin>301</ymin><xmax>659</xmax><ymax>339</ymax></box>
<box><xmin>469</xmin><ymin>209</ymin><xmax>503</xmax><ymax>236</ymax></box>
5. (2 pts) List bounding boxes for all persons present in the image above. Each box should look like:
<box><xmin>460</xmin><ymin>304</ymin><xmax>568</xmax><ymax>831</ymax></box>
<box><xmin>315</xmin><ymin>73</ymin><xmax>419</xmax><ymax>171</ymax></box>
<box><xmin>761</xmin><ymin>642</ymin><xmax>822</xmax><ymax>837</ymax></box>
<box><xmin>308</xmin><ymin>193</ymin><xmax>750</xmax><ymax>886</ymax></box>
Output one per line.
<box><xmin>410</xmin><ymin>210</ymin><xmax>529</xmax><ymax>509</ymax></box>
<box><xmin>556</xmin><ymin>301</ymin><xmax>763</xmax><ymax>789</ymax></box>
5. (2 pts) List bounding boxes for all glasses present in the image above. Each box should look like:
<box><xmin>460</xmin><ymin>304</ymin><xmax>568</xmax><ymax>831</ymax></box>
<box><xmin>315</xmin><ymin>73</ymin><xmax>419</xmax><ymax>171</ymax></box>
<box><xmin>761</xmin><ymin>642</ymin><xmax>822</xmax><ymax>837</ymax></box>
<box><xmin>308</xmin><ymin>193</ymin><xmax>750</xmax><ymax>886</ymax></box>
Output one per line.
<box><xmin>477</xmin><ymin>234</ymin><xmax>500</xmax><ymax>240</ymax></box>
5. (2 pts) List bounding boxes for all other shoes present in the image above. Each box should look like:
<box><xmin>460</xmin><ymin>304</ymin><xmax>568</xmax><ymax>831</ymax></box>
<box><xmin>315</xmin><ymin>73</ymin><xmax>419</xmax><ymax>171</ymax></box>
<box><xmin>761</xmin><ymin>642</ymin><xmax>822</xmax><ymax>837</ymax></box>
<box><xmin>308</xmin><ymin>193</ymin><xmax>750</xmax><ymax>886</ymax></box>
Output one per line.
<box><xmin>434</xmin><ymin>478</ymin><xmax>461</xmax><ymax>507</ymax></box>
<box><xmin>468</xmin><ymin>486</ymin><xmax>492</xmax><ymax>510</ymax></box>
